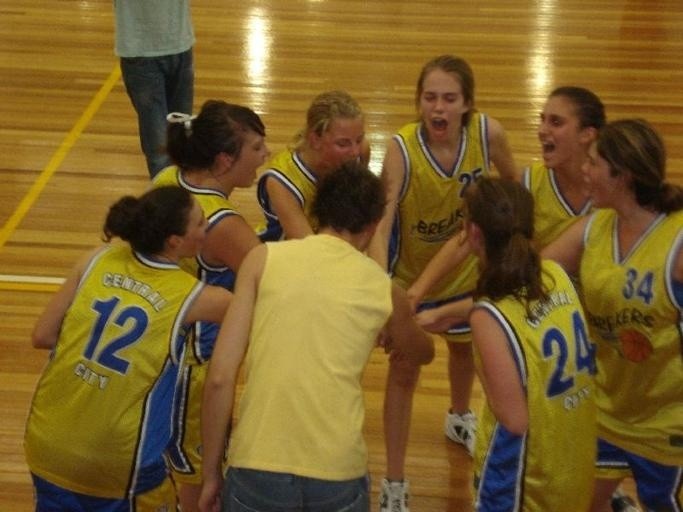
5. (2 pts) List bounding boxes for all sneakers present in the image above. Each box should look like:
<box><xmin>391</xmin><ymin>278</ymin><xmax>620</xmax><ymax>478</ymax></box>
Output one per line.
<box><xmin>379</xmin><ymin>478</ymin><xmax>409</xmax><ymax>512</ymax></box>
<box><xmin>445</xmin><ymin>408</ymin><xmax>480</xmax><ymax>456</ymax></box>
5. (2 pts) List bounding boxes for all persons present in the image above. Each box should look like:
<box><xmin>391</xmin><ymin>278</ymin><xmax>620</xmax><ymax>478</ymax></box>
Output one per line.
<box><xmin>188</xmin><ymin>158</ymin><xmax>435</xmax><ymax>512</ymax></box>
<box><xmin>414</xmin><ymin>176</ymin><xmax>599</xmax><ymax>512</ymax></box>
<box><xmin>144</xmin><ymin>98</ymin><xmax>272</xmax><ymax>512</ymax></box>
<box><xmin>367</xmin><ymin>54</ymin><xmax>518</xmax><ymax>512</ymax></box>
<box><xmin>110</xmin><ymin>0</ymin><xmax>195</xmax><ymax>179</ymax></box>
<box><xmin>415</xmin><ymin>115</ymin><xmax>682</xmax><ymax>512</ymax></box>
<box><xmin>20</xmin><ymin>184</ymin><xmax>234</xmax><ymax>512</ymax></box>
<box><xmin>250</xmin><ymin>88</ymin><xmax>372</xmax><ymax>244</ymax></box>
<box><xmin>402</xmin><ymin>85</ymin><xmax>606</xmax><ymax>312</ymax></box>
